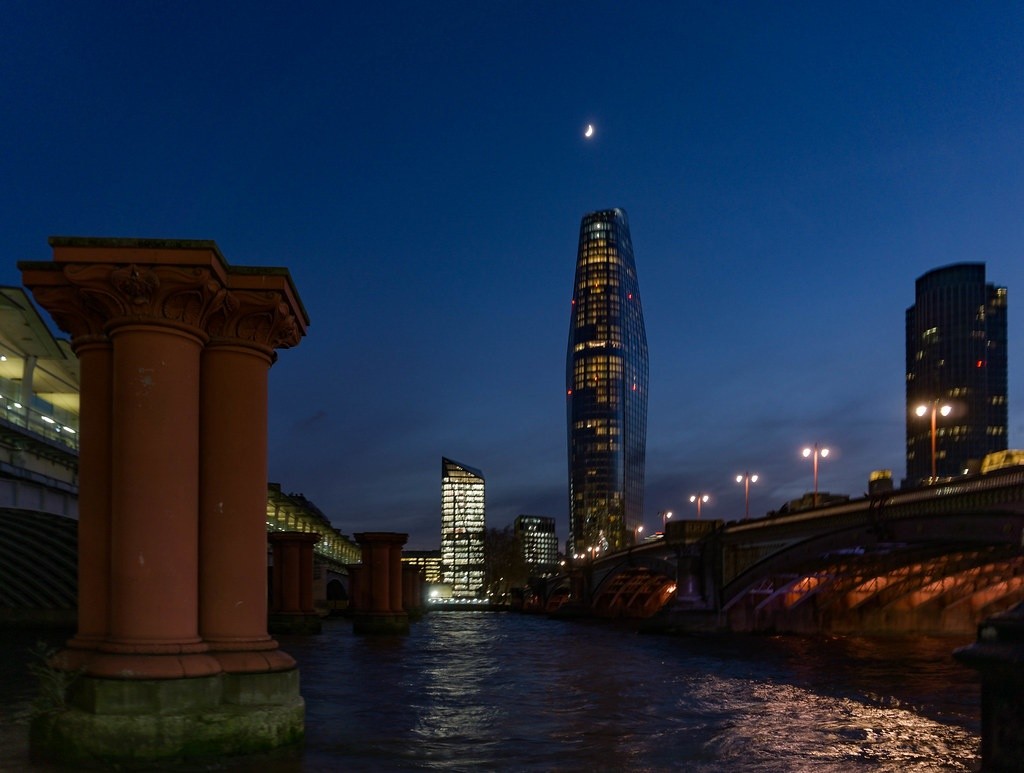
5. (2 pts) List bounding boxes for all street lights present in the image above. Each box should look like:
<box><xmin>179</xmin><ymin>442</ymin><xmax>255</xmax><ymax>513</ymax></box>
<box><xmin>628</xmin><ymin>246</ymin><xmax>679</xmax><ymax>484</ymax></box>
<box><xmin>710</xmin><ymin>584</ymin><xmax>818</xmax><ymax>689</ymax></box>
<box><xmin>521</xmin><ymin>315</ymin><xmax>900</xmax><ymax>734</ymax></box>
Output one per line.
<box><xmin>633</xmin><ymin>526</ymin><xmax>644</xmax><ymax>546</ymax></box>
<box><xmin>588</xmin><ymin>547</ymin><xmax>600</xmax><ymax>559</ymax></box>
<box><xmin>690</xmin><ymin>492</ymin><xmax>709</xmax><ymax>520</ymax></box>
<box><xmin>574</xmin><ymin>554</ymin><xmax>585</xmax><ymax>559</ymax></box>
<box><xmin>802</xmin><ymin>443</ymin><xmax>830</xmax><ymax>506</ymax></box>
<box><xmin>915</xmin><ymin>398</ymin><xmax>952</xmax><ymax>484</ymax></box>
<box><xmin>656</xmin><ymin>512</ymin><xmax>672</xmax><ymax>532</ymax></box>
<box><xmin>736</xmin><ymin>471</ymin><xmax>758</xmax><ymax>521</ymax></box>
